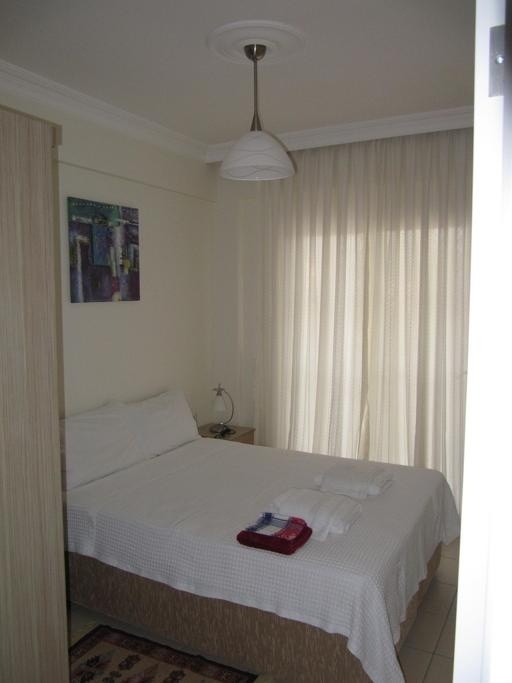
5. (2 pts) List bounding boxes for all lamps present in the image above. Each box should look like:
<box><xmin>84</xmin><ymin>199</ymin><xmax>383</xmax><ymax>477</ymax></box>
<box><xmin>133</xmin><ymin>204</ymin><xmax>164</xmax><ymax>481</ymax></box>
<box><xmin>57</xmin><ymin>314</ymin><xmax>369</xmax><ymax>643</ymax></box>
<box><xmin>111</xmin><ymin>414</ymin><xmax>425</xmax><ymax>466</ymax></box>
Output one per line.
<box><xmin>205</xmin><ymin>17</ymin><xmax>310</xmax><ymax>182</ymax></box>
<box><xmin>210</xmin><ymin>383</ymin><xmax>236</xmax><ymax>439</ymax></box>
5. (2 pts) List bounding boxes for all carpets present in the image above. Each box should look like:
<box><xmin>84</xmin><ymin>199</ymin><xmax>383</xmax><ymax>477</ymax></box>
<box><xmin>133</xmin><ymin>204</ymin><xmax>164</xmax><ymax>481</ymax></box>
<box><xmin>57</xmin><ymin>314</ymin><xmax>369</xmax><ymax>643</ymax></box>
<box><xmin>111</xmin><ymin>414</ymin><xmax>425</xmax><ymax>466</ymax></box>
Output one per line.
<box><xmin>64</xmin><ymin>621</ymin><xmax>258</xmax><ymax>682</ymax></box>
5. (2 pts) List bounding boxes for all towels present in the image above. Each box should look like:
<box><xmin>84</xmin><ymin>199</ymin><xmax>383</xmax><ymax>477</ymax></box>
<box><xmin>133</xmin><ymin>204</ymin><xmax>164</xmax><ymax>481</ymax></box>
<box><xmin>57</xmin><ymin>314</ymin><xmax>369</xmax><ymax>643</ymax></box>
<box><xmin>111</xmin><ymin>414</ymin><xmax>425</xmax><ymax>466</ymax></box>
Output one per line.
<box><xmin>234</xmin><ymin>458</ymin><xmax>395</xmax><ymax>555</ymax></box>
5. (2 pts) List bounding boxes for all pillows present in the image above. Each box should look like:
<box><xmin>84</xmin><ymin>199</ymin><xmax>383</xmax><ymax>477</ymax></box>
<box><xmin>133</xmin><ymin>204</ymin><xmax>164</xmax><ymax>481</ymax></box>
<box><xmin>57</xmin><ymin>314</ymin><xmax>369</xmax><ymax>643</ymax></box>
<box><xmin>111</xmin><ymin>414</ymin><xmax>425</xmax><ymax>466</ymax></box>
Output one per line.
<box><xmin>64</xmin><ymin>388</ymin><xmax>203</xmax><ymax>490</ymax></box>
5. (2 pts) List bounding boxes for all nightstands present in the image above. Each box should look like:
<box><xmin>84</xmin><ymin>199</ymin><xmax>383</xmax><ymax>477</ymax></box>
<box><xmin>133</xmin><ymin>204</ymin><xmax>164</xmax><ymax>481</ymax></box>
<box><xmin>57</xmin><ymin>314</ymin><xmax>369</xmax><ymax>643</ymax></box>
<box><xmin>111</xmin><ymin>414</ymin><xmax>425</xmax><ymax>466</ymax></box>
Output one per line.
<box><xmin>198</xmin><ymin>421</ymin><xmax>257</xmax><ymax>445</ymax></box>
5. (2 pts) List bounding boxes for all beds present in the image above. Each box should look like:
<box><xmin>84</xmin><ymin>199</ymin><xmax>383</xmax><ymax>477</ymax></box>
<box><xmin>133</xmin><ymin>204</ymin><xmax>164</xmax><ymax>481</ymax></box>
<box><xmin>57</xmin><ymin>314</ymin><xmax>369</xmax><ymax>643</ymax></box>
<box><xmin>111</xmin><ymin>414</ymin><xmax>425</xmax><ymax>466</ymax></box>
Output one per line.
<box><xmin>55</xmin><ymin>385</ymin><xmax>458</xmax><ymax>681</ymax></box>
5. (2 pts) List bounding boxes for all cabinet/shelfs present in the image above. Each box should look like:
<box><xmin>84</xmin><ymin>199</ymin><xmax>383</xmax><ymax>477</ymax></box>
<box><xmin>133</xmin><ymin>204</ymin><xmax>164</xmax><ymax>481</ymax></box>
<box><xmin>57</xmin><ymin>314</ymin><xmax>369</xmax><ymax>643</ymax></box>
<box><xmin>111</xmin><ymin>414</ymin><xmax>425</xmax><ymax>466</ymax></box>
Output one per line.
<box><xmin>1</xmin><ymin>102</ymin><xmax>74</xmax><ymax>683</ymax></box>
